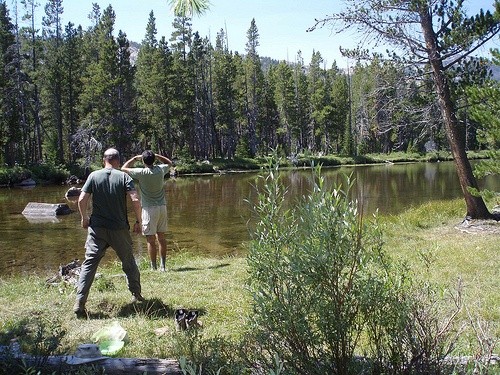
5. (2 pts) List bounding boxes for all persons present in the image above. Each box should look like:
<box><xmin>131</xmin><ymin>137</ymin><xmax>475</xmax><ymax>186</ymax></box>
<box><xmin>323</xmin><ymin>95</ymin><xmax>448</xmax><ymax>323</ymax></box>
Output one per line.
<box><xmin>73</xmin><ymin>148</ymin><xmax>147</xmax><ymax>316</ymax></box>
<box><xmin>319</xmin><ymin>150</ymin><xmax>324</xmax><ymax>167</ymax></box>
<box><xmin>292</xmin><ymin>154</ymin><xmax>298</xmax><ymax>169</ymax></box>
<box><xmin>121</xmin><ymin>150</ymin><xmax>173</xmax><ymax>272</ymax></box>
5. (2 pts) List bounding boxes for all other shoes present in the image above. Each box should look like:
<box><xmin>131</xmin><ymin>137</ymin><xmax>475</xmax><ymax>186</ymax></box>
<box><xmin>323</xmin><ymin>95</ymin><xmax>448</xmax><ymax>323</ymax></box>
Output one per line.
<box><xmin>132</xmin><ymin>293</ymin><xmax>145</xmax><ymax>302</ymax></box>
<box><xmin>74</xmin><ymin>308</ymin><xmax>92</xmax><ymax>318</ymax></box>
<box><xmin>151</xmin><ymin>266</ymin><xmax>165</xmax><ymax>271</ymax></box>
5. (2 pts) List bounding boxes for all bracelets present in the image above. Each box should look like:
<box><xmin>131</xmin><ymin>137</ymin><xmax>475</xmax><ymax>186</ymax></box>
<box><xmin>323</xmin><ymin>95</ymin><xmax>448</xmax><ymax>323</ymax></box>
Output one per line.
<box><xmin>136</xmin><ymin>220</ymin><xmax>141</xmax><ymax>224</ymax></box>
